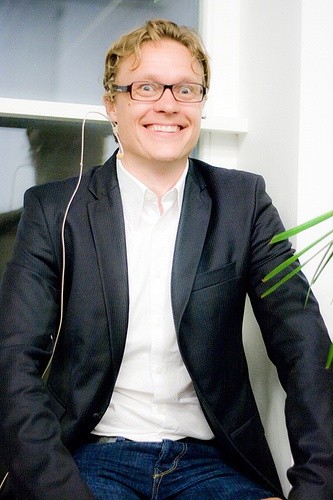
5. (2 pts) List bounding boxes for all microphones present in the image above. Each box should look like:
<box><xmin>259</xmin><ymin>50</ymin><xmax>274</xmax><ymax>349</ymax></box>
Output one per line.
<box><xmin>112</xmin><ymin>127</ymin><xmax>124</xmax><ymax>160</ymax></box>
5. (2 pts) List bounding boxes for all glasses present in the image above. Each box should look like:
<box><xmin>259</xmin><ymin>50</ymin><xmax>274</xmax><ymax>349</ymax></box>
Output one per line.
<box><xmin>111</xmin><ymin>80</ymin><xmax>207</xmax><ymax>103</ymax></box>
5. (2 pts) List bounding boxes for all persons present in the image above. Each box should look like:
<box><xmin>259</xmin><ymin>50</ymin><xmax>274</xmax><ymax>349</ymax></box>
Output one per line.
<box><xmin>0</xmin><ymin>18</ymin><xmax>333</xmax><ymax>500</ymax></box>
